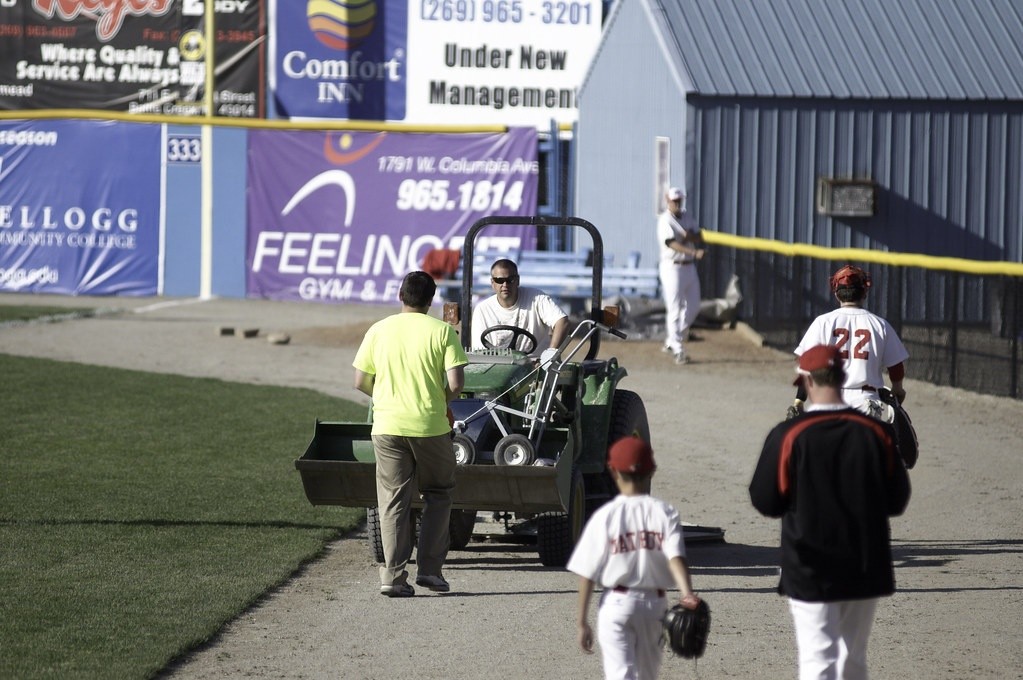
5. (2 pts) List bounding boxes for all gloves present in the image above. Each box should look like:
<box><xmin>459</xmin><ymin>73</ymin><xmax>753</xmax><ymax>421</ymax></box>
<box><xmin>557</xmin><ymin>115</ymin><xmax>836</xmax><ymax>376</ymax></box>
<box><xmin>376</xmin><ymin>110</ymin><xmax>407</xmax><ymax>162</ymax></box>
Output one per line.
<box><xmin>891</xmin><ymin>385</ymin><xmax>906</xmax><ymax>405</ymax></box>
<box><xmin>793</xmin><ymin>399</ymin><xmax>804</xmax><ymax>416</ymax></box>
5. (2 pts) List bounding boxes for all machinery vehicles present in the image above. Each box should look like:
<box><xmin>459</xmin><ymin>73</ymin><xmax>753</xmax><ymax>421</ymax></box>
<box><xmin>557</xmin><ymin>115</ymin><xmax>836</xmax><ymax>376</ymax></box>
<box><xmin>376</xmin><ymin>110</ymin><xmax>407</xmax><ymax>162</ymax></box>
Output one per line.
<box><xmin>293</xmin><ymin>214</ymin><xmax>652</xmax><ymax>569</ymax></box>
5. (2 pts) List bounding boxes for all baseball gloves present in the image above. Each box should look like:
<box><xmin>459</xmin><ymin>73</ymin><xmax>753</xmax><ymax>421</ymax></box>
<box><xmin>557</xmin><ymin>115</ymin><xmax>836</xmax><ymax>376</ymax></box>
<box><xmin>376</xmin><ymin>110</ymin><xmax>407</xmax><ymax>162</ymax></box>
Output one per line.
<box><xmin>655</xmin><ymin>594</ymin><xmax>712</xmax><ymax>669</ymax></box>
<box><xmin>786</xmin><ymin>405</ymin><xmax>807</xmax><ymax>421</ymax></box>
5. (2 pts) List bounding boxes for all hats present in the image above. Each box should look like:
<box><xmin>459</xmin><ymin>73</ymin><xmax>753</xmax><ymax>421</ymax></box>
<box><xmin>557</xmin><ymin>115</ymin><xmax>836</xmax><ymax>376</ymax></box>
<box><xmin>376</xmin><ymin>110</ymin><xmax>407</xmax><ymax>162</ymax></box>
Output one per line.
<box><xmin>667</xmin><ymin>187</ymin><xmax>685</xmax><ymax>201</ymax></box>
<box><xmin>833</xmin><ymin>265</ymin><xmax>867</xmax><ymax>291</ymax></box>
<box><xmin>796</xmin><ymin>345</ymin><xmax>842</xmax><ymax>375</ymax></box>
<box><xmin>608</xmin><ymin>437</ymin><xmax>653</xmax><ymax>473</ymax></box>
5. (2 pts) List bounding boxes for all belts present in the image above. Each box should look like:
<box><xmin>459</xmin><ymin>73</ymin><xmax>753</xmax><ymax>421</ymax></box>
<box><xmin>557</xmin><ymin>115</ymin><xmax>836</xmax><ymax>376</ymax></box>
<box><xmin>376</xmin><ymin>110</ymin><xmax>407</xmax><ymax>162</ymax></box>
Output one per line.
<box><xmin>674</xmin><ymin>260</ymin><xmax>695</xmax><ymax>264</ymax></box>
<box><xmin>612</xmin><ymin>585</ymin><xmax>665</xmax><ymax>598</ymax></box>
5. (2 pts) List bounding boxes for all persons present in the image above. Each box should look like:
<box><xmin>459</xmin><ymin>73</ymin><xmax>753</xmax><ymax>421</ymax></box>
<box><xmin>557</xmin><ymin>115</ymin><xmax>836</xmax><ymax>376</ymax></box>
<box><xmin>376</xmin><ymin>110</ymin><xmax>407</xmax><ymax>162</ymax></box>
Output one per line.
<box><xmin>351</xmin><ymin>270</ymin><xmax>469</xmax><ymax>598</ymax></box>
<box><xmin>794</xmin><ymin>265</ymin><xmax>911</xmax><ymax>426</ymax></box>
<box><xmin>566</xmin><ymin>436</ymin><xmax>711</xmax><ymax>680</ymax></box>
<box><xmin>748</xmin><ymin>345</ymin><xmax>912</xmax><ymax>680</ymax></box>
<box><xmin>656</xmin><ymin>187</ymin><xmax>709</xmax><ymax>365</ymax></box>
<box><xmin>470</xmin><ymin>258</ymin><xmax>571</xmax><ymax>368</ymax></box>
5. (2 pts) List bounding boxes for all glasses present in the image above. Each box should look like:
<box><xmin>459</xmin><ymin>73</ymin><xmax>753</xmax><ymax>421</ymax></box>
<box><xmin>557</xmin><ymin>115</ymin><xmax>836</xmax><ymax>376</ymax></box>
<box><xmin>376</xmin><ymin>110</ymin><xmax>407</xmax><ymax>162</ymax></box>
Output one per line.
<box><xmin>492</xmin><ymin>276</ymin><xmax>516</xmax><ymax>284</ymax></box>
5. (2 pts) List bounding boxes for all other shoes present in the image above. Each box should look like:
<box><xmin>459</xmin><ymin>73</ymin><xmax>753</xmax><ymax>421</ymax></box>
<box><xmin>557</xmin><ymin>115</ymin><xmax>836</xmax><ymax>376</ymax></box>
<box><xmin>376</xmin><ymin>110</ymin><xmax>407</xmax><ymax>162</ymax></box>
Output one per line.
<box><xmin>381</xmin><ymin>581</ymin><xmax>415</xmax><ymax>597</ymax></box>
<box><xmin>673</xmin><ymin>351</ymin><xmax>689</xmax><ymax>365</ymax></box>
<box><xmin>662</xmin><ymin>344</ymin><xmax>677</xmax><ymax>357</ymax></box>
<box><xmin>416</xmin><ymin>570</ymin><xmax>450</xmax><ymax>592</ymax></box>
